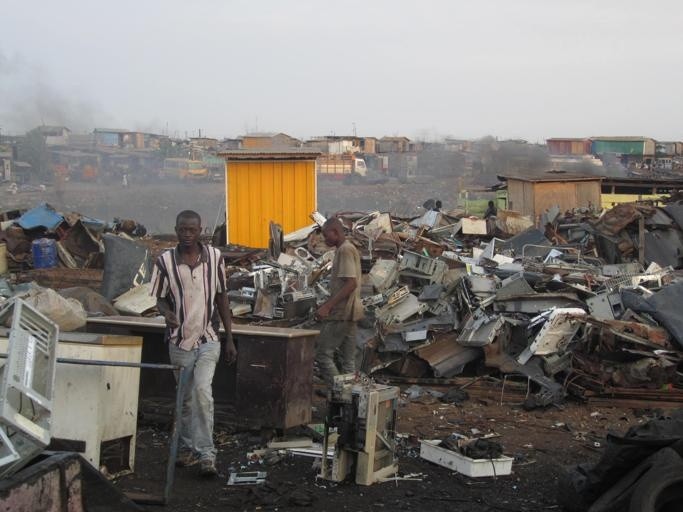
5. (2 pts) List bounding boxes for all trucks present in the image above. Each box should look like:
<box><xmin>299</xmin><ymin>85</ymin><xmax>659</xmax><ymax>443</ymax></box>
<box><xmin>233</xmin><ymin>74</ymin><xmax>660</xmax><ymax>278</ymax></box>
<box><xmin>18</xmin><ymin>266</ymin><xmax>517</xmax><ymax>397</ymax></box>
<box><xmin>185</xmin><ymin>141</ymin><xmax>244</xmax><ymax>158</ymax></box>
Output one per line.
<box><xmin>317</xmin><ymin>159</ymin><xmax>368</xmax><ymax>185</ymax></box>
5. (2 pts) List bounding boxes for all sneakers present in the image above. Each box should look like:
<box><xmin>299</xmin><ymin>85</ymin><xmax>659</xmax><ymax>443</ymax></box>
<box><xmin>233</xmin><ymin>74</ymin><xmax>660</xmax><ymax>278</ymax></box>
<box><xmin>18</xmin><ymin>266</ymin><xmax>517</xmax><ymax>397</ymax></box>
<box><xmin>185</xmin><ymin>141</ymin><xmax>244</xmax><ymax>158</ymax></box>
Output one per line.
<box><xmin>174</xmin><ymin>450</ymin><xmax>200</xmax><ymax>467</ymax></box>
<box><xmin>196</xmin><ymin>456</ymin><xmax>219</xmax><ymax>476</ymax></box>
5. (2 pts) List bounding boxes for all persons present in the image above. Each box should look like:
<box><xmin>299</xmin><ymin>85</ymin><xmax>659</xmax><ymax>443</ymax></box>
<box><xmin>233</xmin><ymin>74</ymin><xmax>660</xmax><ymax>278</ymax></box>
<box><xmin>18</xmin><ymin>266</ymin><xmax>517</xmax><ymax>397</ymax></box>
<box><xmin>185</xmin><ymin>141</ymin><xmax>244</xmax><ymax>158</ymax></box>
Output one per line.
<box><xmin>433</xmin><ymin>200</ymin><xmax>442</xmax><ymax>212</ymax></box>
<box><xmin>150</xmin><ymin>208</ymin><xmax>238</xmax><ymax>478</ymax></box>
<box><xmin>312</xmin><ymin>219</ymin><xmax>363</xmax><ymax>386</ymax></box>
<box><xmin>483</xmin><ymin>200</ymin><xmax>497</xmax><ymax>221</ymax></box>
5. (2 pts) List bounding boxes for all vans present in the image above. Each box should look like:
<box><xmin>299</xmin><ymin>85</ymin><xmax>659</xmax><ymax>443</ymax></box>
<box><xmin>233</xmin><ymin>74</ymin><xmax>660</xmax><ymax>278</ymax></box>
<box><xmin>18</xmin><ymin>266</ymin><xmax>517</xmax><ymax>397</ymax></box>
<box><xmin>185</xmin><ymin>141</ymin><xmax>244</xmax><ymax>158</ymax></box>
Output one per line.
<box><xmin>163</xmin><ymin>158</ymin><xmax>208</xmax><ymax>182</ymax></box>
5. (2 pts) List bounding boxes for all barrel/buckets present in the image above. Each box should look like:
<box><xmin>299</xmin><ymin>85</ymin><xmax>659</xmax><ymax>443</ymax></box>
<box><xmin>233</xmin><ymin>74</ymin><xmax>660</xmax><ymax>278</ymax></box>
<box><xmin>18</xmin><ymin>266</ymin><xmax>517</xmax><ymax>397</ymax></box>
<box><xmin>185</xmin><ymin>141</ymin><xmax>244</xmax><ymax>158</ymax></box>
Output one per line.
<box><xmin>32</xmin><ymin>238</ymin><xmax>57</xmax><ymax>269</ymax></box>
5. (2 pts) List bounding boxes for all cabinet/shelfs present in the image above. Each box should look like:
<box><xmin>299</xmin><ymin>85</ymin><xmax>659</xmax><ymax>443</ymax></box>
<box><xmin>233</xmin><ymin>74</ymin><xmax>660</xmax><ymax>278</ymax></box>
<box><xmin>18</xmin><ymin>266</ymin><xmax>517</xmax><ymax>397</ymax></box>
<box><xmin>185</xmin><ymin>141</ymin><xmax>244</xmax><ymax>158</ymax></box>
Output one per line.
<box><xmin>85</xmin><ymin>322</ymin><xmax>319</xmax><ymax>438</ymax></box>
